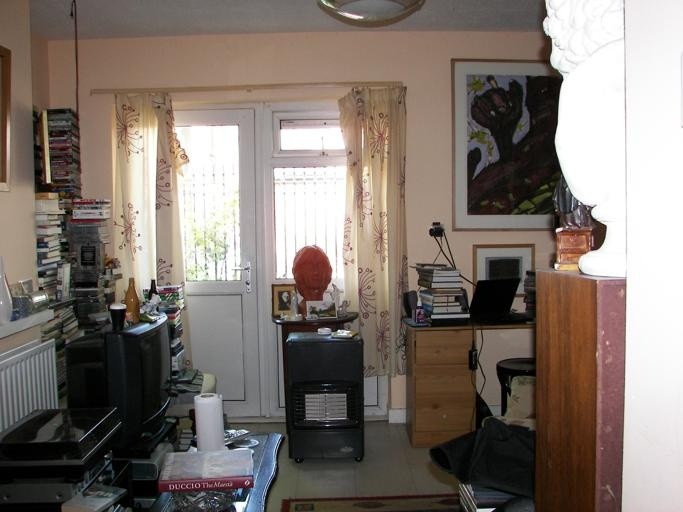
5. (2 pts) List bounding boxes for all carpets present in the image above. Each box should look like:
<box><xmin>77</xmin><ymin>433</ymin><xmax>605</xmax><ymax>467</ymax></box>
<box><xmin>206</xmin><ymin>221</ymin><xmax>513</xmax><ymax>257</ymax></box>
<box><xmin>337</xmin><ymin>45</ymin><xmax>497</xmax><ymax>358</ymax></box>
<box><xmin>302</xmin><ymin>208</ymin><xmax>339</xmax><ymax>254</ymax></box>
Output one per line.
<box><xmin>281</xmin><ymin>493</ymin><xmax>461</xmax><ymax>512</ymax></box>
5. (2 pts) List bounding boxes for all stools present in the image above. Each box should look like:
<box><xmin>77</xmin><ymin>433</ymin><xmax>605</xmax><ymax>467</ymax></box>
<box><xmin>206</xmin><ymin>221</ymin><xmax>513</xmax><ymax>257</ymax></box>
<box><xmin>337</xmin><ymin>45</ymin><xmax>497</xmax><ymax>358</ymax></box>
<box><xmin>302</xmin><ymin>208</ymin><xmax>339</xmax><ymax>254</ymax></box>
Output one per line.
<box><xmin>496</xmin><ymin>356</ymin><xmax>536</xmax><ymax>415</ymax></box>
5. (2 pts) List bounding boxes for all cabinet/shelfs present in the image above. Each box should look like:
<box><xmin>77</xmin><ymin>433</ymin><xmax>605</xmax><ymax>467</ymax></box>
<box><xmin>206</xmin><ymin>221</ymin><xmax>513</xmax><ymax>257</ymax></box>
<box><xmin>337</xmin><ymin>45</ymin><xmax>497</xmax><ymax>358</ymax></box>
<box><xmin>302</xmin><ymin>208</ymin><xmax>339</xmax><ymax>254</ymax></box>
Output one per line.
<box><xmin>414</xmin><ymin>328</ymin><xmax>475</xmax><ymax>449</ymax></box>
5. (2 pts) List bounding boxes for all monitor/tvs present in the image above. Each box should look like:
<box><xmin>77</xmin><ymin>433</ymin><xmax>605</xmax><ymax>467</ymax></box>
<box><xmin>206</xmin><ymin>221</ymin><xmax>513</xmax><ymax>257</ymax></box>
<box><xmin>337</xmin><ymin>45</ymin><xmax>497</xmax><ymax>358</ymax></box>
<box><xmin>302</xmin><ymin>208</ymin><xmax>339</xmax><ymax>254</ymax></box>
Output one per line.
<box><xmin>64</xmin><ymin>313</ymin><xmax>174</xmax><ymax>445</ymax></box>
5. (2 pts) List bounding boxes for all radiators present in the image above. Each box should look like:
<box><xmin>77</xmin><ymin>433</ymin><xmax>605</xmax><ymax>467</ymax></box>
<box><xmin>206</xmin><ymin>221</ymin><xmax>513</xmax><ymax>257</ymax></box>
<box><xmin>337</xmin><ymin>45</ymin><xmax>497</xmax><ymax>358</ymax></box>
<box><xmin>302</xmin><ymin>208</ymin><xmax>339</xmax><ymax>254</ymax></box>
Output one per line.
<box><xmin>0</xmin><ymin>338</ymin><xmax>59</xmax><ymax>432</ymax></box>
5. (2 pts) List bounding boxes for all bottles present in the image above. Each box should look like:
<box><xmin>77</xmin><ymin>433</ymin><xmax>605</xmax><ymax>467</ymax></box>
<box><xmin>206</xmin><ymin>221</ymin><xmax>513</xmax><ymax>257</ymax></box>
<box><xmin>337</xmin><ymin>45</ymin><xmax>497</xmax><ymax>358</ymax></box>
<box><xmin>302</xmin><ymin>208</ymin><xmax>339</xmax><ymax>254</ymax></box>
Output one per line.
<box><xmin>148</xmin><ymin>279</ymin><xmax>160</xmax><ymax>313</ymax></box>
<box><xmin>123</xmin><ymin>277</ymin><xmax>140</xmax><ymax>324</ymax></box>
<box><xmin>416</xmin><ymin>306</ymin><xmax>425</xmax><ymax>324</ymax></box>
<box><xmin>417</xmin><ymin>295</ymin><xmax>422</xmax><ymax>306</ymax></box>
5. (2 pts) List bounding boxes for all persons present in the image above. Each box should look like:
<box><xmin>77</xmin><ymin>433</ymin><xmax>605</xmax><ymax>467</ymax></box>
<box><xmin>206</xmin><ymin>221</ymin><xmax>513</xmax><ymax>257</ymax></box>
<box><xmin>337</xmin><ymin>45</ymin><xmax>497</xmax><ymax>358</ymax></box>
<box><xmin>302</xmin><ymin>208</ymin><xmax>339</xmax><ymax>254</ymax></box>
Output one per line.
<box><xmin>279</xmin><ymin>292</ymin><xmax>291</xmax><ymax>310</ymax></box>
<box><xmin>292</xmin><ymin>244</ymin><xmax>333</xmax><ymax>316</ymax></box>
<box><xmin>310</xmin><ymin>307</ymin><xmax>320</xmax><ymax>319</ymax></box>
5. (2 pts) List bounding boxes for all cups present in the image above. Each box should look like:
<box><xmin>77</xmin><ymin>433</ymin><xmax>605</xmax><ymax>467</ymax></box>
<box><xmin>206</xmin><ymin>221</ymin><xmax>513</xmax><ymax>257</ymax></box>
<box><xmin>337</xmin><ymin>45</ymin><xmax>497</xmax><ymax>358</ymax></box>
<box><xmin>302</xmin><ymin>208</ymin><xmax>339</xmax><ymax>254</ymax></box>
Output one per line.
<box><xmin>10</xmin><ymin>296</ymin><xmax>34</xmax><ymax>321</ymax></box>
<box><xmin>109</xmin><ymin>303</ymin><xmax>127</xmax><ymax>332</ymax></box>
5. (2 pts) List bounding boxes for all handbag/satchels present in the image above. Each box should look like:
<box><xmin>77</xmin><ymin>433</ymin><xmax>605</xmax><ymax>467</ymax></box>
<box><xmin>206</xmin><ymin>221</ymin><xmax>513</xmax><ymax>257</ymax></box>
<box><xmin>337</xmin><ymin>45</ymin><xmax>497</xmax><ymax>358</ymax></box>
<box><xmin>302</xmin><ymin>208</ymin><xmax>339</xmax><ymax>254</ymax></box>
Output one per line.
<box><xmin>464</xmin><ymin>416</ymin><xmax>536</xmax><ymax>499</ymax></box>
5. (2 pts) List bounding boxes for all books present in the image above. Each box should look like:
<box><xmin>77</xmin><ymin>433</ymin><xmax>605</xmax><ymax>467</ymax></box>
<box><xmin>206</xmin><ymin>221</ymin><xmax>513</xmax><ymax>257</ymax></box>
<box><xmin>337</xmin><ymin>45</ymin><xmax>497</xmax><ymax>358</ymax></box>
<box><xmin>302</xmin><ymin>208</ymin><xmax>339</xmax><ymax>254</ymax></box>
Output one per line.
<box><xmin>157</xmin><ymin>449</ymin><xmax>254</xmax><ymax>492</ymax></box>
<box><xmin>144</xmin><ymin>285</ymin><xmax>185</xmax><ymax>358</ymax></box>
<box><xmin>61</xmin><ymin>481</ymin><xmax>128</xmax><ymax>512</ymax></box>
<box><xmin>32</xmin><ymin>109</ymin><xmax>123</xmax><ymax>343</ymax></box>
<box><xmin>524</xmin><ymin>269</ymin><xmax>535</xmax><ymax>325</ymax></box>
<box><xmin>410</xmin><ymin>262</ymin><xmax>471</xmax><ymax>320</ymax></box>
<box><xmin>332</xmin><ymin>330</ymin><xmax>358</xmax><ymax>339</ymax></box>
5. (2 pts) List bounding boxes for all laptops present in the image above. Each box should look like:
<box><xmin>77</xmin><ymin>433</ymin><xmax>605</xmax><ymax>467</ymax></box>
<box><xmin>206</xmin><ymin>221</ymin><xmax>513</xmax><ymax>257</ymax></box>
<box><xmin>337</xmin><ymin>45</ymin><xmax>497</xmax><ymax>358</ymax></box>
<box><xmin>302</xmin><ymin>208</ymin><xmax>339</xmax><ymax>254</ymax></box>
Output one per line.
<box><xmin>469</xmin><ymin>276</ymin><xmax>525</xmax><ymax>326</ymax></box>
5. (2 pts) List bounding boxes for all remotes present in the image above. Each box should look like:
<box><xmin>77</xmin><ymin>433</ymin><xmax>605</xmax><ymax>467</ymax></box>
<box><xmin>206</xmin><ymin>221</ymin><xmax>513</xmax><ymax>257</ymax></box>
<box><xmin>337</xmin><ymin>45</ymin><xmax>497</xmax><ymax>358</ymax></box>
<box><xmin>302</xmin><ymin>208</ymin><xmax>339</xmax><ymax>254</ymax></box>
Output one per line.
<box><xmin>222</xmin><ymin>429</ymin><xmax>250</xmax><ymax>443</ymax></box>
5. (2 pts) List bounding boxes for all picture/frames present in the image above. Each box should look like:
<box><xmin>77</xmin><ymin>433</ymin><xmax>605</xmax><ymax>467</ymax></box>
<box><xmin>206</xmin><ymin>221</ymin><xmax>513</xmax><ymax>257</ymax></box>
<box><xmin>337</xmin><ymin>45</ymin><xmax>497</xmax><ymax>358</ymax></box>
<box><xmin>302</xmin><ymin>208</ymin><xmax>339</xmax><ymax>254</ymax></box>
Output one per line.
<box><xmin>450</xmin><ymin>59</ymin><xmax>558</xmax><ymax>232</ymax></box>
<box><xmin>272</xmin><ymin>283</ymin><xmax>296</xmax><ymax>315</ymax></box>
<box><xmin>471</xmin><ymin>244</ymin><xmax>535</xmax><ymax>297</ymax></box>
<box><xmin>0</xmin><ymin>45</ymin><xmax>12</xmax><ymax>191</ymax></box>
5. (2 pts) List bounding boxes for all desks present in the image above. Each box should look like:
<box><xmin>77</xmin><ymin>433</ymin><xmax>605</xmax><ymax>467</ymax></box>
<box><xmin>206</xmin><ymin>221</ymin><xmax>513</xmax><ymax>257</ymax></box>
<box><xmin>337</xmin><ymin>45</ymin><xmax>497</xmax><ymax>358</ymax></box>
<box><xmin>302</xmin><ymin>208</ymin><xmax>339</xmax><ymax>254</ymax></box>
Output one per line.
<box><xmin>266</xmin><ymin>310</ymin><xmax>358</xmax><ymax>334</ymax></box>
<box><xmin>535</xmin><ymin>267</ymin><xmax>626</xmax><ymax>512</ymax></box>
<box><xmin>148</xmin><ymin>432</ymin><xmax>286</xmax><ymax>512</ymax></box>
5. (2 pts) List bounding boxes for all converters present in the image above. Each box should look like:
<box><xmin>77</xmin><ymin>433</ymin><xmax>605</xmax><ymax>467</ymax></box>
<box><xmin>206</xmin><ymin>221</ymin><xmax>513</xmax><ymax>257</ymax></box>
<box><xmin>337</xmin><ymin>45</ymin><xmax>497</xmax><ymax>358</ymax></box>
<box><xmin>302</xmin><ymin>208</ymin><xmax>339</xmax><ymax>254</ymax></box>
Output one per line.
<box><xmin>468</xmin><ymin>349</ymin><xmax>478</xmax><ymax>372</ymax></box>
